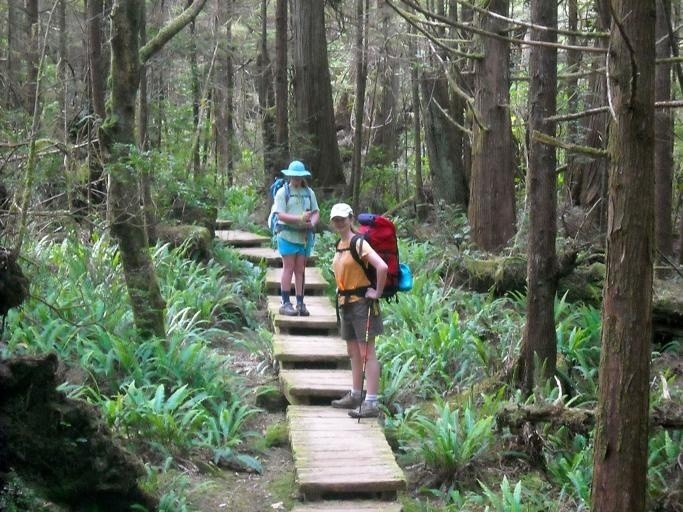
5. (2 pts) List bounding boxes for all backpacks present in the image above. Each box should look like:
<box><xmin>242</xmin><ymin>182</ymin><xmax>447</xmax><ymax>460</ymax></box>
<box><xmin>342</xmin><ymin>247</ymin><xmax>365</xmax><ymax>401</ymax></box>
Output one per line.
<box><xmin>335</xmin><ymin>214</ymin><xmax>413</xmax><ymax>297</ymax></box>
<box><xmin>268</xmin><ymin>179</ymin><xmax>312</xmax><ymax>232</ymax></box>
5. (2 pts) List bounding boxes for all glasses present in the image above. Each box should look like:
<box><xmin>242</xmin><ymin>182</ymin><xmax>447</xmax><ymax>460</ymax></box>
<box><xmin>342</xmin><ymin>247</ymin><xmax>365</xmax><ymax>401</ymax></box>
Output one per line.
<box><xmin>333</xmin><ymin>218</ymin><xmax>345</xmax><ymax>223</ymax></box>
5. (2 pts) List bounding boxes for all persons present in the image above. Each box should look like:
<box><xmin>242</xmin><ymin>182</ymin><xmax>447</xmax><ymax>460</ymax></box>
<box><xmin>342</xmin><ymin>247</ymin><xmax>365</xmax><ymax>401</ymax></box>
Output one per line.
<box><xmin>328</xmin><ymin>201</ymin><xmax>389</xmax><ymax>418</ymax></box>
<box><xmin>265</xmin><ymin>160</ymin><xmax>320</xmax><ymax>317</ymax></box>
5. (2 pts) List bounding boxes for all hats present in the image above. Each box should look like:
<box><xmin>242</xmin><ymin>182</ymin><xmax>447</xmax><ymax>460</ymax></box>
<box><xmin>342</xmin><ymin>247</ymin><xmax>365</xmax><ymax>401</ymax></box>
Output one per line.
<box><xmin>330</xmin><ymin>203</ymin><xmax>353</xmax><ymax>220</ymax></box>
<box><xmin>281</xmin><ymin>160</ymin><xmax>311</xmax><ymax>176</ymax></box>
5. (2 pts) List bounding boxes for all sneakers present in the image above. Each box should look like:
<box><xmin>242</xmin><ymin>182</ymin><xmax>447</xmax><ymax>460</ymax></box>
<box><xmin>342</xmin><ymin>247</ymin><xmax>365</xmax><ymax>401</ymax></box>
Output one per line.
<box><xmin>331</xmin><ymin>392</ymin><xmax>380</xmax><ymax>417</ymax></box>
<box><xmin>279</xmin><ymin>302</ymin><xmax>310</xmax><ymax>316</ymax></box>
